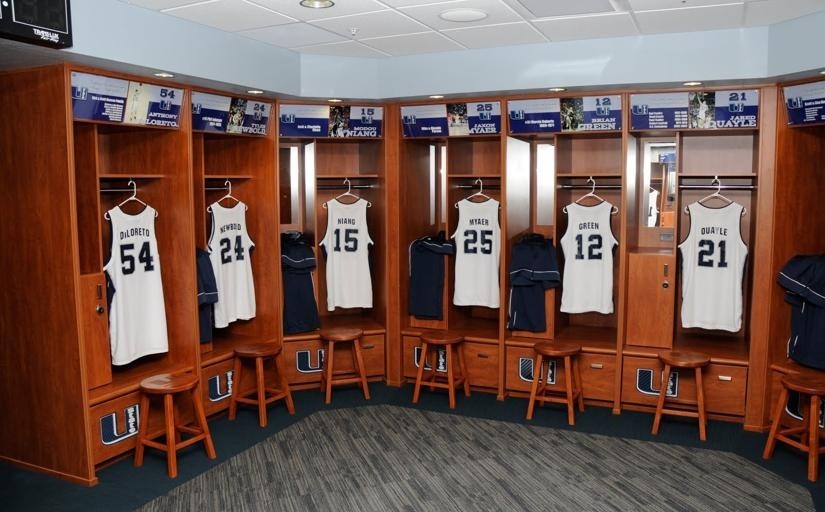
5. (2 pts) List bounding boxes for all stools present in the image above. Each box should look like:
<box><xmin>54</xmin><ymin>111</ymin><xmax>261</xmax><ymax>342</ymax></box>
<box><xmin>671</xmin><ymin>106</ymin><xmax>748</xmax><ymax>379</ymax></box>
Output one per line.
<box><xmin>653</xmin><ymin>350</ymin><xmax>712</xmax><ymax>442</ymax></box>
<box><xmin>412</xmin><ymin>329</ymin><xmax>470</xmax><ymax>410</ymax></box>
<box><xmin>761</xmin><ymin>369</ymin><xmax>823</xmax><ymax>484</ymax></box>
<box><xmin>525</xmin><ymin>340</ymin><xmax>585</xmax><ymax>426</ymax></box>
<box><xmin>135</xmin><ymin>372</ymin><xmax>216</xmax><ymax>480</ymax></box>
<box><xmin>319</xmin><ymin>326</ymin><xmax>370</xmax><ymax>399</ymax></box>
<box><xmin>230</xmin><ymin>342</ymin><xmax>296</xmax><ymax>427</ymax></box>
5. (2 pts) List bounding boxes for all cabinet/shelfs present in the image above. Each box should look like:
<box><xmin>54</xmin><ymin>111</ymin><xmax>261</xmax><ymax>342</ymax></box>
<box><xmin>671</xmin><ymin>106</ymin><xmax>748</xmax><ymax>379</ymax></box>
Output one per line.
<box><xmin>275</xmin><ymin>95</ymin><xmax>387</xmax><ymax>397</ymax></box>
<box><xmin>391</xmin><ymin>94</ymin><xmax>503</xmax><ymax>400</ymax></box>
<box><xmin>187</xmin><ymin>82</ymin><xmax>280</xmax><ymax>420</ymax></box>
<box><xmin>503</xmin><ymin>92</ymin><xmax>622</xmax><ymax>410</ymax></box>
<box><xmin>2</xmin><ymin>62</ymin><xmax>199</xmax><ymax>486</ymax></box>
<box><xmin>766</xmin><ymin>71</ymin><xmax>825</xmax><ymax>432</ymax></box>
<box><xmin>623</xmin><ymin>89</ymin><xmax>761</xmax><ymax>427</ymax></box>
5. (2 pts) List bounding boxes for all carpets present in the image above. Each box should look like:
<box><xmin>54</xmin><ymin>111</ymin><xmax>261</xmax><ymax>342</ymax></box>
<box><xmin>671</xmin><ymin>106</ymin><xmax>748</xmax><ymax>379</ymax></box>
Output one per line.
<box><xmin>137</xmin><ymin>403</ymin><xmax>816</xmax><ymax>512</ymax></box>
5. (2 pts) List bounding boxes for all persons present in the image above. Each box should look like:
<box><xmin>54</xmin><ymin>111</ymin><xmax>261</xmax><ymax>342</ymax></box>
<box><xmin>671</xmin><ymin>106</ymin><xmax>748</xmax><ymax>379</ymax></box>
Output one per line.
<box><xmin>328</xmin><ymin>105</ymin><xmax>351</xmax><ymax>138</ymax></box>
<box><xmin>560</xmin><ymin>98</ymin><xmax>584</xmax><ymax>132</ymax></box>
<box><xmin>689</xmin><ymin>92</ymin><xmax>716</xmax><ymax>131</ymax></box>
<box><xmin>446</xmin><ymin>102</ymin><xmax>468</xmax><ymax>133</ymax></box>
<box><xmin>225</xmin><ymin>102</ymin><xmax>246</xmax><ymax>134</ymax></box>
